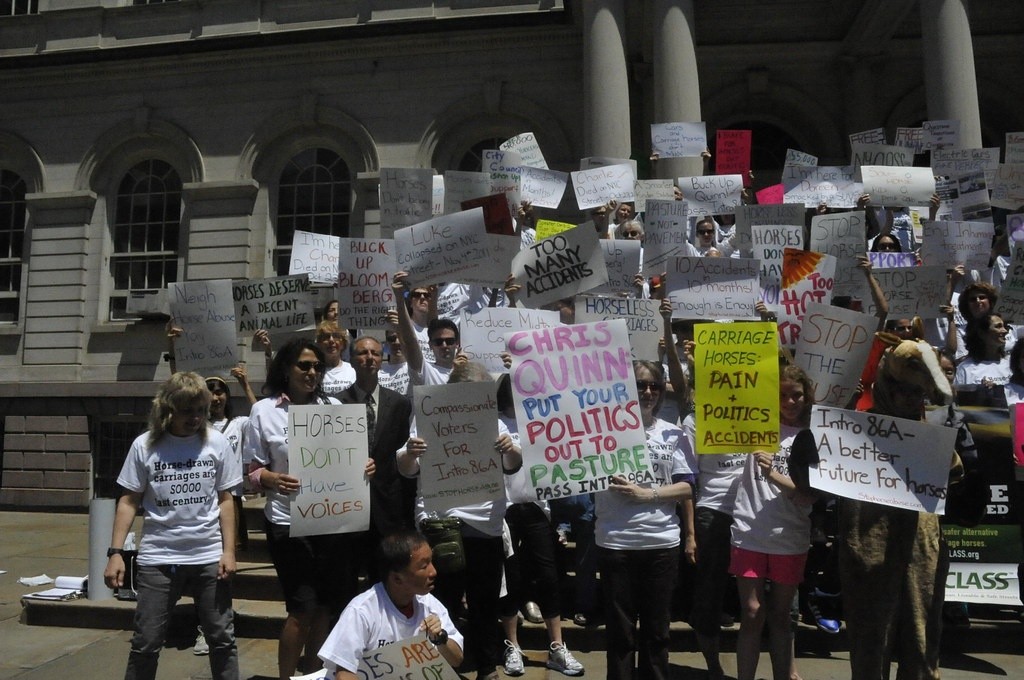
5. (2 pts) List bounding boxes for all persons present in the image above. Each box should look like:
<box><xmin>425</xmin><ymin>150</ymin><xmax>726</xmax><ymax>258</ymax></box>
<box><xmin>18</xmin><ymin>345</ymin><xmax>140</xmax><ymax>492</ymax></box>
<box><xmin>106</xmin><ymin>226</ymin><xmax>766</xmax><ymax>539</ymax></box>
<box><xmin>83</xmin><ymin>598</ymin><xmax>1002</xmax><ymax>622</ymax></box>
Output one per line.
<box><xmin>635</xmin><ymin>145</ymin><xmax>1024</xmax><ymax>680</ymax></box>
<box><xmin>194</xmin><ymin>368</ymin><xmax>257</xmax><ymax>657</ymax></box>
<box><xmin>166</xmin><ymin>199</ymin><xmax>697</xmax><ymax>680</ymax></box>
<box><xmin>104</xmin><ymin>370</ymin><xmax>241</xmax><ymax>680</ymax></box>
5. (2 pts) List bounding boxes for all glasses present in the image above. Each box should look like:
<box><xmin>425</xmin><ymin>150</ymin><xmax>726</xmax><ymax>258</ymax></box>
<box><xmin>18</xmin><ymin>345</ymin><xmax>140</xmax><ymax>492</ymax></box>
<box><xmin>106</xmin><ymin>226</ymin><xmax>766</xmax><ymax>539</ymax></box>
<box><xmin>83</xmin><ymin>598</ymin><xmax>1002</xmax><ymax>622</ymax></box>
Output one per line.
<box><xmin>878</xmin><ymin>243</ymin><xmax>896</xmax><ymax>249</ymax></box>
<box><xmin>431</xmin><ymin>338</ymin><xmax>456</xmax><ymax>346</ymax></box>
<box><xmin>386</xmin><ymin>336</ymin><xmax>399</xmax><ymax>342</ymax></box>
<box><xmin>622</xmin><ymin>231</ymin><xmax>639</xmax><ymax>237</ymax></box>
<box><xmin>637</xmin><ymin>380</ymin><xmax>660</xmax><ymax>391</ymax></box>
<box><xmin>697</xmin><ymin>229</ymin><xmax>714</xmax><ymax>234</ymax></box>
<box><xmin>294</xmin><ymin>362</ymin><xmax>326</xmax><ymax>373</ymax></box>
<box><xmin>411</xmin><ymin>292</ymin><xmax>428</xmax><ymax>298</ymax></box>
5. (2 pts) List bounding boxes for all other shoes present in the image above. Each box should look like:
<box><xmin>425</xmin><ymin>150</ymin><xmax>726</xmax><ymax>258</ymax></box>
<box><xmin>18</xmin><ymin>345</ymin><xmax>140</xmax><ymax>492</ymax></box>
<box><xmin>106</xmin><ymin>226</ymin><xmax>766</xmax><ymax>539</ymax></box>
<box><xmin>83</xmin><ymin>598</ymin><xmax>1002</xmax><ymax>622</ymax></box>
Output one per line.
<box><xmin>573</xmin><ymin>614</ymin><xmax>588</xmax><ymax>625</ymax></box>
<box><xmin>557</xmin><ymin>528</ymin><xmax>568</xmax><ymax>545</ymax></box>
<box><xmin>521</xmin><ymin>601</ymin><xmax>544</xmax><ymax>622</ymax></box>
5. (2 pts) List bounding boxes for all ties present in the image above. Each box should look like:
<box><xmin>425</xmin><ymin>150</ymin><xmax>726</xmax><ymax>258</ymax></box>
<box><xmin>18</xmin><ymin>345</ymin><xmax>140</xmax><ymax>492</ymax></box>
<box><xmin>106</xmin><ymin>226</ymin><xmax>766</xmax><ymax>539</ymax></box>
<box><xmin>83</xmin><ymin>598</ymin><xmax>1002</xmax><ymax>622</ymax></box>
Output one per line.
<box><xmin>364</xmin><ymin>394</ymin><xmax>376</xmax><ymax>459</ymax></box>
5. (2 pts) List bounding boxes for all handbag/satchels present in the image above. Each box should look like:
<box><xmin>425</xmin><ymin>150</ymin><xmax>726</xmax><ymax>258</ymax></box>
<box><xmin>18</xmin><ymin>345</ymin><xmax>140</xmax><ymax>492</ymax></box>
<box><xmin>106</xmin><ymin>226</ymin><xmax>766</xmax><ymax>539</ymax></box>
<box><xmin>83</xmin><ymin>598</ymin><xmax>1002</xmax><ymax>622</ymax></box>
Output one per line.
<box><xmin>420</xmin><ymin>517</ymin><xmax>465</xmax><ymax>575</ymax></box>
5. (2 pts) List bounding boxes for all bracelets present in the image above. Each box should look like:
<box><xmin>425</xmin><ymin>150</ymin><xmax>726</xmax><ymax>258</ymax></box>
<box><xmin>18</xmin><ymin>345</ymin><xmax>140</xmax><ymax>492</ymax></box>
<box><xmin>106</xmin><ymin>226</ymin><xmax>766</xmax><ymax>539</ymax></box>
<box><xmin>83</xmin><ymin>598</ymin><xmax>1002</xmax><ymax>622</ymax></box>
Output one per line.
<box><xmin>429</xmin><ymin>628</ymin><xmax>448</xmax><ymax>645</ymax></box>
<box><xmin>107</xmin><ymin>547</ymin><xmax>124</xmax><ymax>558</ymax></box>
<box><xmin>652</xmin><ymin>489</ymin><xmax>660</xmax><ymax>505</ymax></box>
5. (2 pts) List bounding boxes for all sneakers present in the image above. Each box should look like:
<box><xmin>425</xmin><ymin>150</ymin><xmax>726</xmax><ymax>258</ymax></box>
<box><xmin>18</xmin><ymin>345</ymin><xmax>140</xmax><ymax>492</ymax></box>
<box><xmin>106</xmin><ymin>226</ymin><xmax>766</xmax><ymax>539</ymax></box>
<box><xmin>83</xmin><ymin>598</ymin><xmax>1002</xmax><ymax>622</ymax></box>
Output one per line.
<box><xmin>807</xmin><ymin>597</ymin><xmax>839</xmax><ymax>633</ymax></box>
<box><xmin>193</xmin><ymin>626</ymin><xmax>235</xmax><ymax>654</ymax></box>
<box><xmin>502</xmin><ymin>639</ymin><xmax>525</xmax><ymax>675</ymax></box>
<box><xmin>547</xmin><ymin>641</ymin><xmax>585</xmax><ymax>675</ymax></box>
